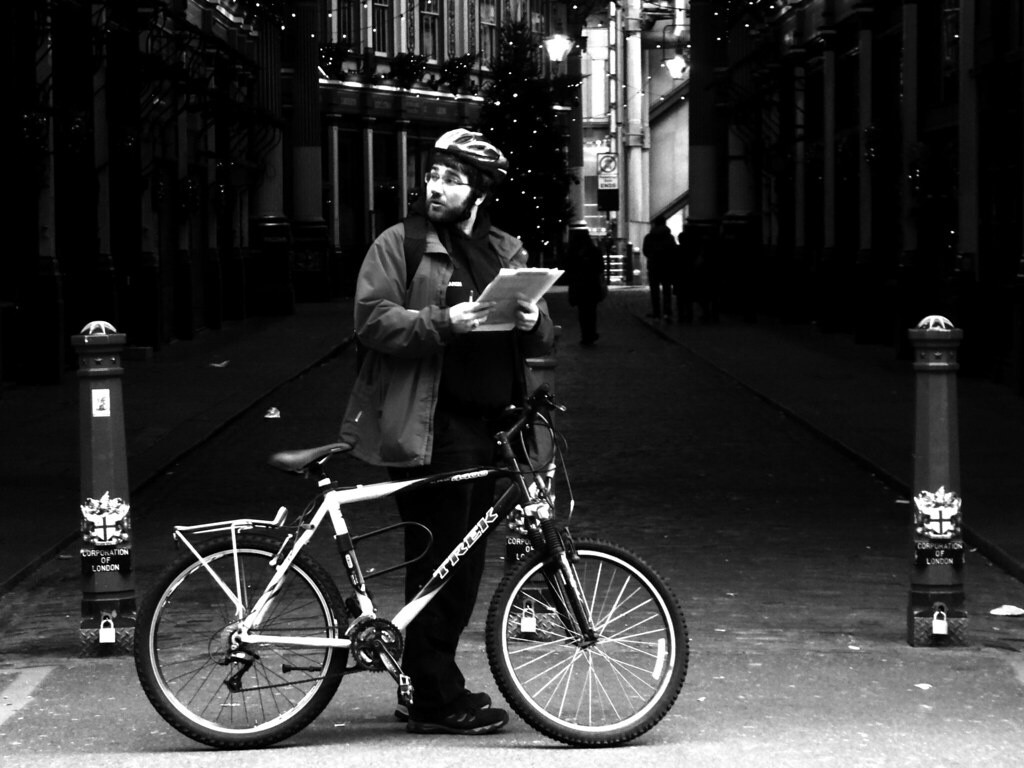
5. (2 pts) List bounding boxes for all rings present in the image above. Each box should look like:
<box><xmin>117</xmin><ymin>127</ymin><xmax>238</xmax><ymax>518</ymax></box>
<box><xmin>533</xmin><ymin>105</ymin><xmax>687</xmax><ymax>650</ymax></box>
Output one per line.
<box><xmin>472</xmin><ymin>319</ymin><xmax>479</xmax><ymax>328</ymax></box>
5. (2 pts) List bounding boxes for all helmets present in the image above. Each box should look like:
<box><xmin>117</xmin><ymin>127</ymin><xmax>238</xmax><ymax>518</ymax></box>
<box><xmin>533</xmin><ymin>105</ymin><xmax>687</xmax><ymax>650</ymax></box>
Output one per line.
<box><xmin>433</xmin><ymin>127</ymin><xmax>509</xmax><ymax>183</ymax></box>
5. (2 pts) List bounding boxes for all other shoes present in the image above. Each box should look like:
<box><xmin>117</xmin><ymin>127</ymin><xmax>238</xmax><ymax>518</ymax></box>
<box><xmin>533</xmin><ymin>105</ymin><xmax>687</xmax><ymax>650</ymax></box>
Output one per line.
<box><xmin>647</xmin><ymin>312</ymin><xmax>661</xmax><ymax>318</ymax></box>
<box><xmin>664</xmin><ymin>315</ymin><xmax>674</xmax><ymax>325</ymax></box>
<box><xmin>581</xmin><ymin>334</ymin><xmax>599</xmax><ymax>347</ymax></box>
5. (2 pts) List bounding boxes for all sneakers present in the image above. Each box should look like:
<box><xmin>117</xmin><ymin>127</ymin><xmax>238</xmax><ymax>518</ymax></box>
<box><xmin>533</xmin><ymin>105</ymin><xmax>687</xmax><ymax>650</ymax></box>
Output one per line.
<box><xmin>395</xmin><ymin>689</ymin><xmax>492</xmax><ymax>725</ymax></box>
<box><xmin>406</xmin><ymin>705</ymin><xmax>509</xmax><ymax>735</ymax></box>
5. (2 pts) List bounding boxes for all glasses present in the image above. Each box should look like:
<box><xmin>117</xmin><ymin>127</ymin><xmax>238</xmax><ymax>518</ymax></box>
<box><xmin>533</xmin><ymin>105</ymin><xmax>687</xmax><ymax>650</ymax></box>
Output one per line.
<box><xmin>425</xmin><ymin>171</ymin><xmax>475</xmax><ymax>186</ymax></box>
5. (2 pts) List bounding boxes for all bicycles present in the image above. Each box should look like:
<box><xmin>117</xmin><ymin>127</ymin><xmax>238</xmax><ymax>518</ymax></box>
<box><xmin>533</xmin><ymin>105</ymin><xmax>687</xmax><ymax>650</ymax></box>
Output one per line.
<box><xmin>133</xmin><ymin>357</ymin><xmax>692</xmax><ymax>748</ymax></box>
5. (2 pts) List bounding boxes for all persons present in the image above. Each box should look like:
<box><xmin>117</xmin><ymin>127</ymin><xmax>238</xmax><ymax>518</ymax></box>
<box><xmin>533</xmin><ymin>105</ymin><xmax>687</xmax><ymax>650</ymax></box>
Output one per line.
<box><xmin>567</xmin><ymin>216</ymin><xmax>724</xmax><ymax>347</ymax></box>
<box><xmin>337</xmin><ymin>126</ymin><xmax>553</xmax><ymax>734</ymax></box>
